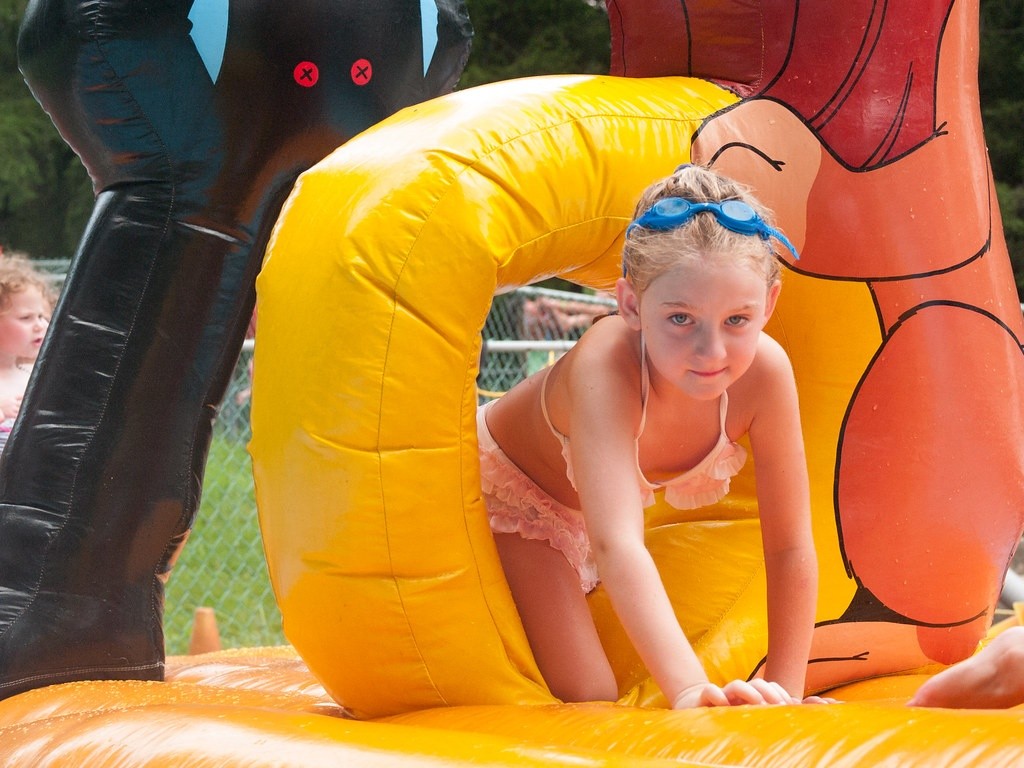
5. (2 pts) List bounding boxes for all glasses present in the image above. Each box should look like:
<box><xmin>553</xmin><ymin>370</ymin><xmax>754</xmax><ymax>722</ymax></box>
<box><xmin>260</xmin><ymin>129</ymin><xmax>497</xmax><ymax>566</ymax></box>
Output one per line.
<box><xmin>623</xmin><ymin>197</ymin><xmax>799</xmax><ymax>279</ymax></box>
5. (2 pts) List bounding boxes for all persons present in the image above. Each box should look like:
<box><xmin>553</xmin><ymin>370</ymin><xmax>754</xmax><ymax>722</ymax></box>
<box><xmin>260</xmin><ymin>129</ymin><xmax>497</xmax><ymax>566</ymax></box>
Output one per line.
<box><xmin>905</xmin><ymin>624</ymin><xmax>1024</xmax><ymax>711</ymax></box>
<box><xmin>0</xmin><ymin>251</ymin><xmax>55</xmax><ymax>456</ymax></box>
<box><xmin>474</xmin><ymin>159</ymin><xmax>854</xmax><ymax>710</ymax></box>
